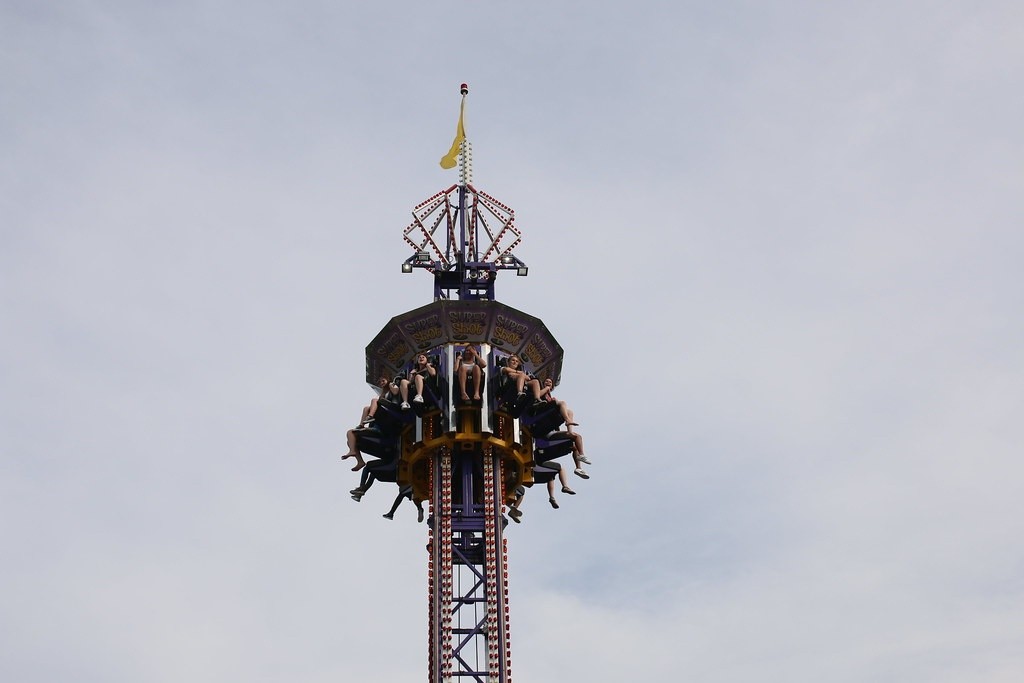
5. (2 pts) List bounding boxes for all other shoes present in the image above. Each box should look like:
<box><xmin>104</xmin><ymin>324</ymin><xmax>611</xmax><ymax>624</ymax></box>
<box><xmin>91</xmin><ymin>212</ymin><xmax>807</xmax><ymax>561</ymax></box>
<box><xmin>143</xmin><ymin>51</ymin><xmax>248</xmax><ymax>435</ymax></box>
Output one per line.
<box><xmin>383</xmin><ymin>513</ymin><xmax>393</xmax><ymax>521</ymax></box>
<box><xmin>400</xmin><ymin>401</ymin><xmax>411</xmax><ymax>410</ymax></box>
<box><xmin>510</xmin><ymin>506</ymin><xmax>523</xmax><ymax>517</ymax></box>
<box><xmin>473</xmin><ymin>395</ymin><xmax>481</xmax><ymax>401</ymax></box>
<box><xmin>461</xmin><ymin>392</ymin><xmax>470</xmax><ymax>401</ymax></box>
<box><xmin>508</xmin><ymin>511</ymin><xmax>521</xmax><ymax>523</ymax></box>
<box><xmin>350</xmin><ymin>488</ymin><xmax>365</xmax><ymax>494</ymax></box>
<box><xmin>363</xmin><ymin>415</ymin><xmax>375</xmax><ymax>423</ymax></box>
<box><xmin>533</xmin><ymin>398</ymin><xmax>547</xmax><ymax>408</ymax></box>
<box><xmin>353</xmin><ymin>425</ymin><xmax>366</xmax><ymax>432</ymax></box>
<box><xmin>413</xmin><ymin>394</ymin><xmax>424</xmax><ymax>404</ymax></box>
<box><xmin>351</xmin><ymin>495</ymin><xmax>360</xmax><ymax>502</ymax></box>
<box><xmin>517</xmin><ymin>392</ymin><xmax>527</xmax><ymax>401</ymax></box>
<box><xmin>549</xmin><ymin>496</ymin><xmax>560</xmax><ymax>509</ymax></box>
<box><xmin>561</xmin><ymin>487</ymin><xmax>577</xmax><ymax>495</ymax></box>
<box><xmin>417</xmin><ymin>507</ymin><xmax>425</xmax><ymax>522</ymax></box>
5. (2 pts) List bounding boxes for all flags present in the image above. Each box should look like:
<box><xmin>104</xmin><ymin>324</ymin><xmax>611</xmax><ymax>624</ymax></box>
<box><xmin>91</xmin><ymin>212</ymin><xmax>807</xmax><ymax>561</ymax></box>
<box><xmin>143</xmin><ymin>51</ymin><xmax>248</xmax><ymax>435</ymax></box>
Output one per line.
<box><xmin>440</xmin><ymin>102</ymin><xmax>465</xmax><ymax>169</ymax></box>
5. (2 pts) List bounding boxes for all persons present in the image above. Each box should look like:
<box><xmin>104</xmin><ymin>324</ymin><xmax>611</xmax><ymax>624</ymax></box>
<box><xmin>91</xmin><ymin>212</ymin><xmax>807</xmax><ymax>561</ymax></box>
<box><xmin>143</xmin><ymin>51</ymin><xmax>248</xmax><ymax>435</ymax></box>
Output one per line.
<box><xmin>498</xmin><ymin>356</ymin><xmax>547</xmax><ymax>408</ymax></box>
<box><xmin>400</xmin><ymin>353</ymin><xmax>436</xmax><ymax>410</ymax></box>
<box><xmin>383</xmin><ymin>492</ymin><xmax>424</xmax><ymax>523</ymax></box>
<box><xmin>355</xmin><ymin>376</ymin><xmax>399</xmax><ymax>429</ymax></box>
<box><xmin>454</xmin><ymin>344</ymin><xmax>487</xmax><ymax>400</ymax></box>
<box><xmin>341</xmin><ymin>422</ymin><xmax>381</xmax><ymax>472</ymax></box>
<box><xmin>539</xmin><ymin>377</ymin><xmax>578</xmax><ymax>438</ymax></box>
<box><xmin>350</xmin><ymin>459</ymin><xmax>385</xmax><ymax>502</ymax></box>
<box><xmin>534</xmin><ymin>448</ymin><xmax>576</xmax><ymax>509</ymax></box>
<box><xmin>546</xmin><ymin>429</ymin><xmax>591</xmax><ymax>479</ymax></box>
<box><xmin>508</xmin><ymin>495</ymin><xmax>523</xmax><ymax>523</ymax></box>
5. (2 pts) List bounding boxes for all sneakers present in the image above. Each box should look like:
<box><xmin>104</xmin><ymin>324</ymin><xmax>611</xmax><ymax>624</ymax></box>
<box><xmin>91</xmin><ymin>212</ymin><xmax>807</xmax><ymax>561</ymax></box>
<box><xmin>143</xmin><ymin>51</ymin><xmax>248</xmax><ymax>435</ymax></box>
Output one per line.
<box><xmin>574</xmin><ymin>468</ymin><xmax>590</xmax><ymax>479</ymax></box>
<box><xmin>576</xmin><ymin>454</ymin><xmax>591</xmax><ymax>466</ymax></box>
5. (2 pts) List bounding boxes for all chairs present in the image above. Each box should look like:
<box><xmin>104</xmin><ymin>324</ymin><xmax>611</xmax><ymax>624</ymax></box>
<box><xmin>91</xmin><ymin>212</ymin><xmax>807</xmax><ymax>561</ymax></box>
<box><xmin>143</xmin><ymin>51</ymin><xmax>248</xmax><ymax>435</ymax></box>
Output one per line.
<box><xmin>355</xmin><ymin>348</ymin><xmax>573</xmax><ymax>502</ymax></box>
